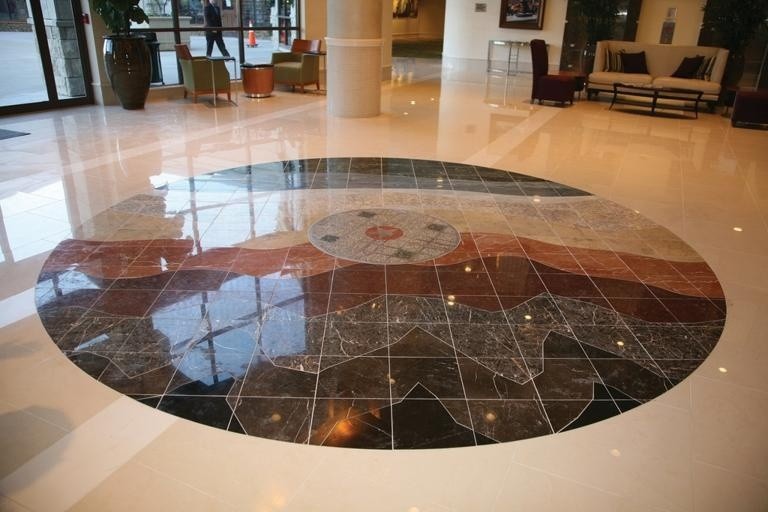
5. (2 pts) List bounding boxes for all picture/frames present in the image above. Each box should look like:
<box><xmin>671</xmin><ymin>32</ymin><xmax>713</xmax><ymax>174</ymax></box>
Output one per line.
<box><xmin>499</xmin><ymin>1</ymin><xmax>544</xmax><ymax>30</ymax></box>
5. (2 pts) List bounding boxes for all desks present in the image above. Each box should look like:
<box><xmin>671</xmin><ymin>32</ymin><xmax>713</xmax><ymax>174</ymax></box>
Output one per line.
<box><xmin>205</xmin><ymin>57</ymin><xmax>238</xmax><ymax>107</ymax></box>
<box><xmin>484</xmin><ymin>72</ymin><xmax>534</xmax><ymax>111</ymax></box>
<box><xmin>240</xmin><ymin>64</ymin><xmax>274</xmax><ymax>98</ymax></box>
<box><xmin>487</xmin><ymin>40</ymin><xmax>550</xmax><ymax>77</ymax></box>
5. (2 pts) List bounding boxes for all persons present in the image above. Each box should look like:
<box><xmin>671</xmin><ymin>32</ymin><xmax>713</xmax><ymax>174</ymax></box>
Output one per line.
<box><xmin>202</xmin><ymin>0</ymin><xmax>232</xmax><ymax>61</ymax></box>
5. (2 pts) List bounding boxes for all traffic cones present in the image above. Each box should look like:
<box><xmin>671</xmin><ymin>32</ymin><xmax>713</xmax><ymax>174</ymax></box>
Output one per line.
<box><xmin>246</xmin><ymin>20</ymin><xmax>260</xmax><ymax>47</ymax></box>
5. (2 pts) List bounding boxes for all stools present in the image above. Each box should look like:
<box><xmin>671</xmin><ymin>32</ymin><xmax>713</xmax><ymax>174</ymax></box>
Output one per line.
<box><xmin>571</xmin><ymin>75</ymin><xmax>584</xmax><ymax>99</ymax></box>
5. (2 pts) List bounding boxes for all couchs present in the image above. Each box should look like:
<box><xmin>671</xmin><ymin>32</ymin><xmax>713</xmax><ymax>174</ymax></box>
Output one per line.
<box><xmin>587</xmin><ymin>40</ymin><xmax>731</xmax><ymax>113</ymax></box>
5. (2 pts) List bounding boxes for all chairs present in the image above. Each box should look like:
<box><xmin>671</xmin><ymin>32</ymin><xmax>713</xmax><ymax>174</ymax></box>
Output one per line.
<box><xmin>528</xmin><ymin>39</ymin><xmax>574</xmax><ymax>107</ymax></box>
<box><xmin>730</xmin><ymin>62</ymin><xmax>768</xmax><ymax>126</ymax></box>
<box><xmin>271</xmin><ymin>39</ymin><xmax>322</xmax><ymax>92</ymax></box>
<box><xmin>173</xmin><ymin>45</ymin><xmax>230</xmax><ymax>104</ymax></box>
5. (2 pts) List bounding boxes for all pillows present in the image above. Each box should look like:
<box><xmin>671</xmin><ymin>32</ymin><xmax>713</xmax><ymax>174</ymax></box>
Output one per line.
<box><xmin>603</xmin><ymin>46</ymin><xmax>648</xmax><ymax>74</ymax></box>
<box><xmin>670</xmin><ymin>54</ymin><xmax>715</xmax><ymax>80</ymax></box>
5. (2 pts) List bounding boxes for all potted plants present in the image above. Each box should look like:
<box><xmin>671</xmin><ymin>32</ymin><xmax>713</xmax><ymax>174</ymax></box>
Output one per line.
<box><xmin>91</xmin><ymin>0</ymin><xmax>153</xmax><ymax>110</ymax></box>
